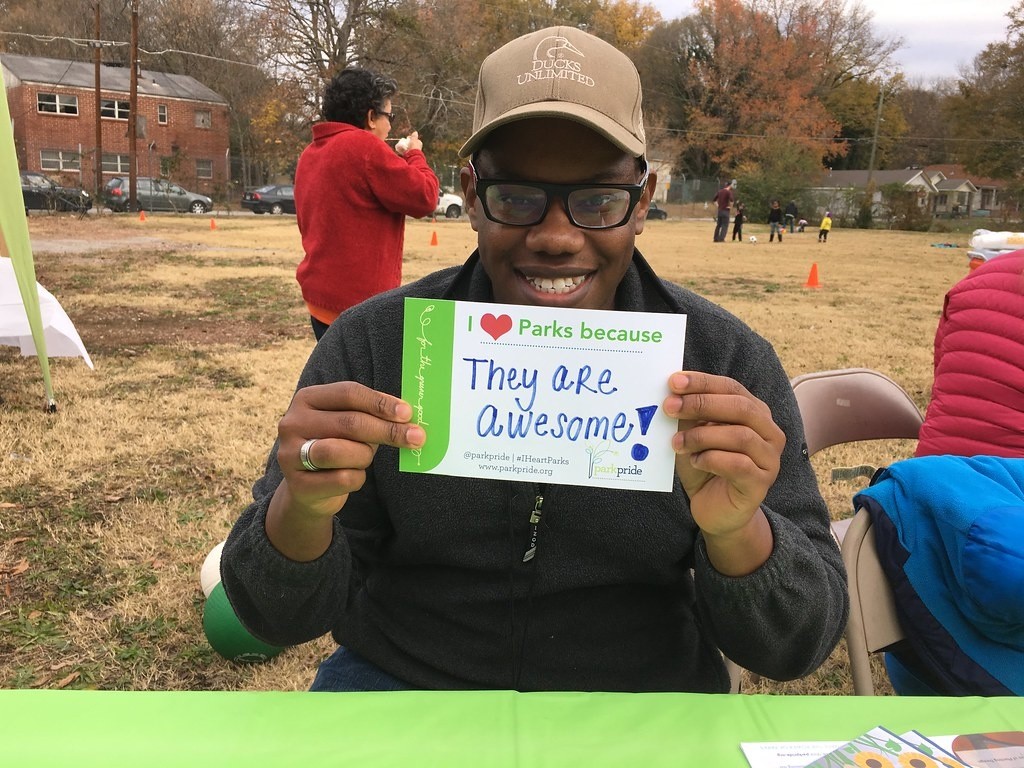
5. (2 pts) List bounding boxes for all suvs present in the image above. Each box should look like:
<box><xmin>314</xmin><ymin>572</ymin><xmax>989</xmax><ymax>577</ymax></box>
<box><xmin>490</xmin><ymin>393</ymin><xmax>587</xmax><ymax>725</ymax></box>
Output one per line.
<box><xmin>102</xmin><ymin>175</ymin><xmax>214</xmax><ymax>214</ymax></box>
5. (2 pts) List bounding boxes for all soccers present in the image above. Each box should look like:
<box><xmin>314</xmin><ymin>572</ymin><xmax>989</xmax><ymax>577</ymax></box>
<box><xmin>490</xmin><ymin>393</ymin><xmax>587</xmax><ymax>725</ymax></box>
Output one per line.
<box><xmin>749</xmin><ymin>236</ymin><xmax>757</xmax><ymax>243</ymax></box>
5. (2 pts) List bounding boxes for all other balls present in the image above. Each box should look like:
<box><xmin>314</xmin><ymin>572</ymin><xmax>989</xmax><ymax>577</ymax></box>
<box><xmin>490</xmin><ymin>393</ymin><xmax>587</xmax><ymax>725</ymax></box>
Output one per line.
<box><xmin>796</xmin><ymin>227</ymin><xmax>801</xmax><ymax>232</ymax></box>
<box><xmin>780</xmin><ymin>229</ymin><xmax>786</xmax><ymax>234</ymax></box>
<box><xmin>199</xmin><ymin>538</ymin><xmax>288</xmax><ymax>666</ymax></box>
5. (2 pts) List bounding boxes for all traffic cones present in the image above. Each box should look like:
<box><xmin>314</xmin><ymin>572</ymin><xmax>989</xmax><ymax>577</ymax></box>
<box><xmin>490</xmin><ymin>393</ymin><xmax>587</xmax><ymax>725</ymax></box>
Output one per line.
<box><xmin>800</xmin><ymin>263</ymin><xmax>824</xmax><ymax>288</ymax></box>
<box><xmin>138</xmin><ymin>210</ymin><xmax>146</xmax><ymax>221</ymax></box>
<box><xmin>210</xmin><ymin>218</ymin><xmax>216</xmax><ymax>230</ymax></box>
<box><xmin>430</xmin><ymin>230</ymin><xmax>439</xmax><ymax>246</ymax></box>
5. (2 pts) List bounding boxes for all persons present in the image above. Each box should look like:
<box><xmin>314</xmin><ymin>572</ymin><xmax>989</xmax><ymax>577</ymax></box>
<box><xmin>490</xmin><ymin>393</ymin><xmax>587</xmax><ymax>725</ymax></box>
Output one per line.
<box><xmin>220</xmin><ymin>25</ymin><xmax>850</xmax><ymax>694</ymax></box>
<box><xmin>885</xmin><ymin>248</ymin><xmax>1024</xmax><ymax>698</ymax></box>
<box><xmin>713</xmin><ymin>182</ymin><xmax>734</xmax><ymax>242</ymax></box>
<box><xmin>782</xmin><ymin>200</ymin><xmax>798</xmax><ymax>233</ymax></box>
<box><xmin>798</xmin><ymin>218</ymin><xmax>807</xmax><ymax>232</ymax></box>
<box><xmin>293</xmin><ymin>68</ymin><xmax>439</xmax><ymax>343</ymax></box>
<box><xmin>818</xmin><ymin>211</ymin><xmax>832</xmax><ymax>243</ymax></box>
<box><xmin>732</xmin><ymin>209</ymin><xmax>744</xmax><ymax>241</ymax></box>
<box><xmin>767</xmin><ymin>200</ymin><xmax>784</xmax><ymax>242</ymax></box>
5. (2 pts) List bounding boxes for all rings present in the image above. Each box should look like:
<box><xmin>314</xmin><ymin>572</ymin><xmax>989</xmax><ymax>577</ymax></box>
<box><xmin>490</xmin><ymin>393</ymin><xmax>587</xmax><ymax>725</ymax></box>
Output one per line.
<box><xmin>300</xmin><ymin>439</ymin><xmax>318</xmax><ymax>472</ymax></box>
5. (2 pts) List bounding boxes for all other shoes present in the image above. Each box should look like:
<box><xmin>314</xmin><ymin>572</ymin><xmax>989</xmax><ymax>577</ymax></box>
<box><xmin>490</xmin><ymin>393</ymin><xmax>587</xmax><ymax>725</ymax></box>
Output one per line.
<box><xmin>818</xmin><ymin>240</ymin><xmax>821</xmax><ymax>242</ymax></box>
<box><xmin>823</xmin><ymin>240</ymin><xmax>826</xmax><ymax>242</ymax></box>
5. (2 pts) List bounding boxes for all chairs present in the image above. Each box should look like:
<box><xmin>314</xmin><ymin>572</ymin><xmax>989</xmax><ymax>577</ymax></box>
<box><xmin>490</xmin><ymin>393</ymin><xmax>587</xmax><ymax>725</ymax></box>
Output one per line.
<box><xmin>843</xmin><ymin>506</ymin><xmax>911</xmax><ymax>694</ymax></box>
<box><xmin>784</xmin><ymin>369</ymin><xmax>921</xmax><ymax>552</ymax></box>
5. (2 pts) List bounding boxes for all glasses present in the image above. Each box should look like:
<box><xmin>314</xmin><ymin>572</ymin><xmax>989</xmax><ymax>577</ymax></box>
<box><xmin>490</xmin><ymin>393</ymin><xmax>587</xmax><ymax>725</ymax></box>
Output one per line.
<box><xmin>468</xmin><ymin>159</ymin><xmax>657</xmax><ymax>230</ymax></box>
<box><xmin>380</xmin><ymin>112</ymin><xmax>395</xmax><ymax>122</ymax></box>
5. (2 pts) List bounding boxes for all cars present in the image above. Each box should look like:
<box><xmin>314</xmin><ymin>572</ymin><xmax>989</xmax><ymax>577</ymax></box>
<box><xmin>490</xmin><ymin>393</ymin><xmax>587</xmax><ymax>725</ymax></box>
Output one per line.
<box><xmin>427</xmin><ymin>186</ymin><xmax>463</xmax><ymax>219</ymax></box>
<box><xmin>240</xmin><ymin>183</ymin><xmax>297</xmax><ymax>215</ymax></box>
<box><xmin>19</xmin><ymin>170</ymin><xmax>93</xmax><ymax>216</ymax></box>
<box><xmin>646</xmin><ymin>200</ymin><xmax>668</xmax><ymax>221</ymax></box>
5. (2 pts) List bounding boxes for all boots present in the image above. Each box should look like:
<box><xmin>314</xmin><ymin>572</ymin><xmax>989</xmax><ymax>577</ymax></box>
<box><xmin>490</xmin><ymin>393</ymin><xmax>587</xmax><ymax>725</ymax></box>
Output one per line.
<box><xmin>769</xmin><ymin>234</ymin><xmax>774</xmax><ymax>241</ymax></box>
<box><xmin>778</xmin><ymin>234</ymin><xmax>782</xmax><ymax>242</ymax></box>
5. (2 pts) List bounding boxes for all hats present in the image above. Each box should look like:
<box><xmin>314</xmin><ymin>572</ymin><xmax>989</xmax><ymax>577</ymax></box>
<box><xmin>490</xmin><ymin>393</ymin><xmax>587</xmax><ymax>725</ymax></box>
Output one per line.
<box><xmin>460</xmin><ymin>26</ymin><xmax>647</xmax><ymax>160</ymax></box>
